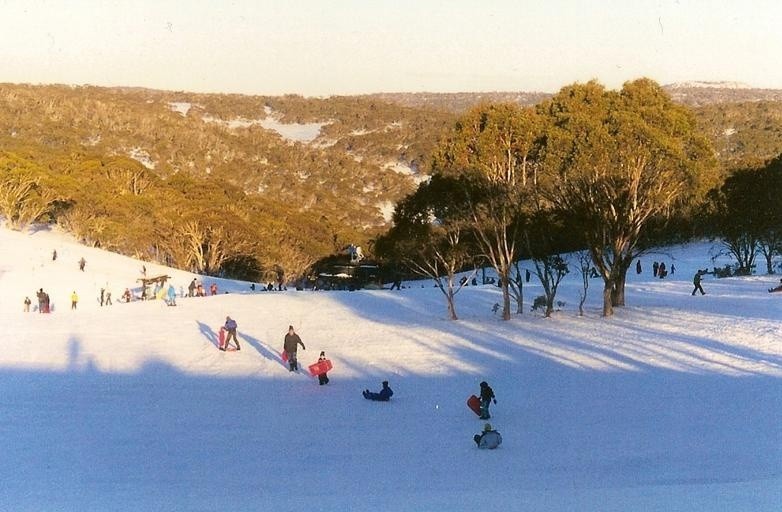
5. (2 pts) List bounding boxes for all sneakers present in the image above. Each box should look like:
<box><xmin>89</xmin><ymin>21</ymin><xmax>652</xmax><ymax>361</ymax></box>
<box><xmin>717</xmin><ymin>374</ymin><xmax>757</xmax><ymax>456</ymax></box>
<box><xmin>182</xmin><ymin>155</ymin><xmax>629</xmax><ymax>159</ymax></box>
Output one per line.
<box><xmin>219</xmin><ymin>348</ymin><xmax>222</xmax><ymax>350</ymax></box>
<box><xmin>486</xmin><ymin>416</ymin><xmax>490</xmax><ymax>419</ymax></box>
<box><xmin>225</xmin><ymin>347</ymin><xmax>227</xmax><ymax>349</ymax></box>
<box><xmin>289</xmin><ymin>369</ymin><xmax>294</xmax><ymax>371</ymax></box>
<box><xmin>320</xmin><ymin>383</ymin><xmax>324</xmax><ymax>385</ymax></box>
<box><xmin>479</xmin><ymin>417</ymin><xmax>485</xmax><ymax>419</ymax></box>
<box><xmin>363</xmin><ymin>391</ymin><xmax>368</xmax><ymax>399</ymax></box>
<box><xmin>295</xmin><ymin>366</ymin><xmax>297</xmax><ymax>370</ymax></box>
<box><xmin>223</xmin><ymin>348</ymin><xmax>226</xmax><ymax>351</ymax></box>
<box><xmin>325</xmin><ymin>379</ymin><xmax>329</xmax><ymax>384</ymax></box>
<box><xmin>237</xmin><ymin>348</ymin><xmax>240</xmax><ymax>350</ymax></box>
<box><xmin>366</xmin><ymin>389</ymin><xmax>370</xmax><ymax>396</ymax></box>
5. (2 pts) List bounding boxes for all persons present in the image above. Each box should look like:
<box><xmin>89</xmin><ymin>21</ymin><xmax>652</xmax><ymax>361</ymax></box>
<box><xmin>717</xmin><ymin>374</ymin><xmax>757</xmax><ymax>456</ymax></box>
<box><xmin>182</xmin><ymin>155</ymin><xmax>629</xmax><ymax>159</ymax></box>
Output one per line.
<box><xmin>460</xmin><ymin>276</ymin><xmax>468</xmax><ymax>286</ymax></box>
<box><xmin>250</xmin><ymin>284</ymin><xmax>255</xmax><ymax>291</ymax></box>
<box><xmin>168</xmin><ymin>285</ymin><xmax>176</xmax><ymax>306</ymax></box>
<box><xmin>486</xmin><ymin>276</ymin><xmax>502</xmax><ymax>287</ymax></box>
<box><xmin>473</xmin><ymin>422</ymin><xmax>502</xmax><ymax>449</ymax></box>
<box><xmin>143</xmin><ymin>285</ymin><xmax>151</xmax><ymax>301</ymax></box>
<box><xmin>692</xmin><ymin>270</ymin><xmax>705</xmax><ymax>296</ymax></box>
<box><xmin>79</xmin><ymin>258</ymin><xmax>87</xmax><ymax>271</ymax></box>
<box><xmin>283</xmin><ymin>324</ymin><xmax>306</xmax><ymax>372</ymax></box>
<box><xmin>362</xmin><ymin>380</ymin><xmax>393</xmax><ymax>401</ymax></box>
<box><xmin>477</xmin><ymin>380</ymin><xmax>498</xmax><ymax>419</ymax></box>
<box><xmin>123</xmin><ymin>288</ymin><xmax>130</xmax><ymax>301</ymax></box>
<box><xmin>670</xmin><ymin>264</ymin><xmax>676</xmax><ymax>276</ymax></box>
<box><xmin>268</xmin><ymin>282</ymin><xmax>273</xmax><ymax>291</ymax></box>
<box><xmin>526</xmin><ymin>269</ymin><xmax>530</xmax><ymax>282</ymax></box>
<box><xmin>142</xmin><ymin>265</ymin><xmax>146</xmax><ymax>275</ymax></box>
<box><xmin>24</xmin><ymin>288</ymin><xmax>49</xmax><ymax>313</ymax></box>
<box><xmin>636</xmin><ymin>260</ymin><xmax>642</xmax><ymax>274</ymax></box>
<box><xmin>52</xmin><ymin>249</ymin><xmax>58</xmax><ymax>261</ymax></box>
<box><xmin>188</xmin><ymin>278</ymin><xmax>205</xmax><ymax>297</ymax></box>
<box><xmin>71</xmin><ymin>291</ymin><xmax>77</xmax><ymax>309</ymax></box>
<box><xmin>222</xmin><ymin>315</ymin><xmax>241</xmax><ymax>352</ymax></box>
<box><xmin>100</xmin><ymin>288</ymin><xmax>112</xmax><ymax>306</ymax></box>
<box><xmin>653</xmin><ymin>262</ymin><xmax>668</xmax><ymax>279</ymax></box>
<box><xmin>210</xmin><ymin>283</ymin><xmax>217</xmax><ymax>297</ymax></box>
<box><xmin>316</xmin><ymin>350</ymin><xmax>330</xmax><ymax>385</ymax></box>
<box><xmin>472</xmin><ymin>278</ymin><xmax>478</xmax><ymax>286</ymax></box>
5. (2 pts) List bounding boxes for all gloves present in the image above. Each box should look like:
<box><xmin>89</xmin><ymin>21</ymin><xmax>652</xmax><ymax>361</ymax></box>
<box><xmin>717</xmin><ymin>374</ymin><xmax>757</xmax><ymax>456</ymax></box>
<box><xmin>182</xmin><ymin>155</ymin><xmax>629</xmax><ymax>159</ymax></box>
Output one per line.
<box><xmin>493</xmin><ymin>399</ymin><xmax>496</xmax><ymax>403</ymax></box>
<box><xmin>303</xmin><ymin>347</ymin><xmax>305</xmax><ymax>349</ymax></box>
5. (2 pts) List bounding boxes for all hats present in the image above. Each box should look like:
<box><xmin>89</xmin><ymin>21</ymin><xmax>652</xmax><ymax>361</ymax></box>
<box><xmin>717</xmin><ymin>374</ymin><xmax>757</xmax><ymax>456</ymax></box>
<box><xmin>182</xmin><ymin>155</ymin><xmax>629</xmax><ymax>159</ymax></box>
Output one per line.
<box><xmin>383</xmin><ymin>381</ymin><xmax>388</xmax><ymax>385</ymax></box>
<box><xmin>485</xmin><ymin>423</ymin><xmax>491</xmax><ymax>429</ymax></box>
<box><xmin>289</xmin><ymin>326</ymin><xmax>293</xmax><ymax>329</ymax></box>
<box><xmin>320</xmin><ymin>352</ymin><xmax>325</xmax><ymax>355</ymax></box>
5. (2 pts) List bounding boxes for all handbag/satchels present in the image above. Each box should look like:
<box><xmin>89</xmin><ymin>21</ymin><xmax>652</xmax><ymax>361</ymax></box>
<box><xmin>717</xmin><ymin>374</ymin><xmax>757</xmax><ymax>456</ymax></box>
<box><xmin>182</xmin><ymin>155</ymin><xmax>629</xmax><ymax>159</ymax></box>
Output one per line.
<box><xmin>282</xmin><ymin>350</ymin><xmax>288</xmax><ymax>361</ymax></box>
